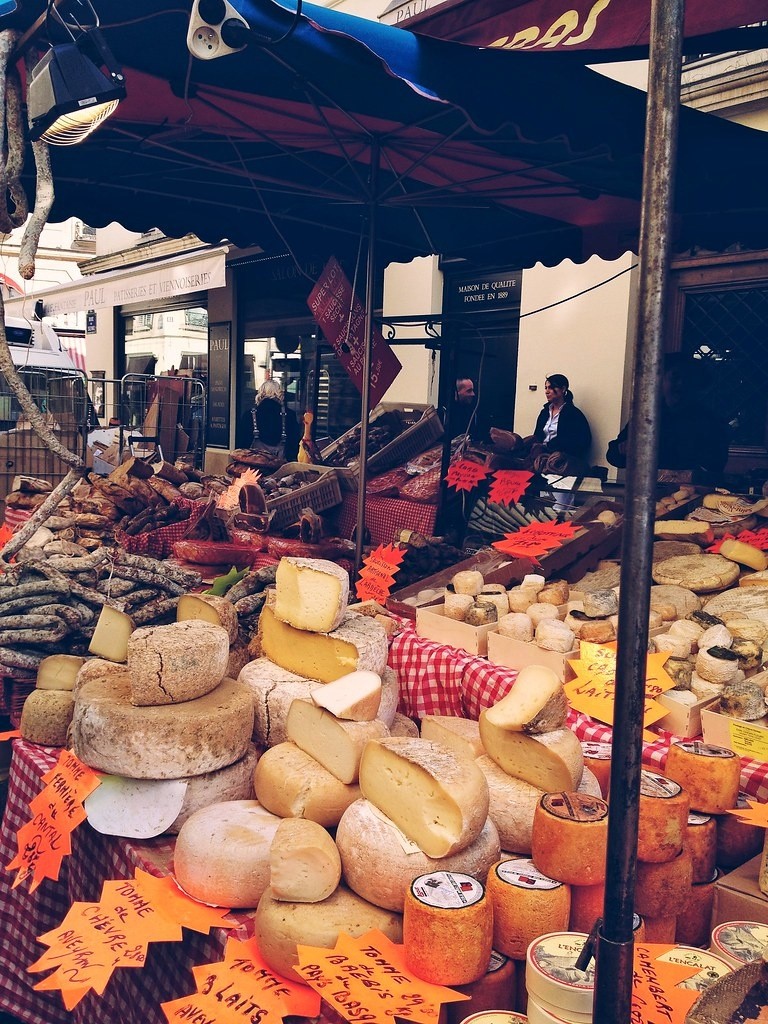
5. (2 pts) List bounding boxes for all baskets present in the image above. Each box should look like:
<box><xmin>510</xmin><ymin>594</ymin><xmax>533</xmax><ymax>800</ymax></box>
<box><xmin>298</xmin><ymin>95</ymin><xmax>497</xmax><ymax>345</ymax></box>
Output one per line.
<box><xmin>264</xmin><ymin>462</ymin><xmax>342</xmax><ymax>531</ymax></box>
<box><xmin>314</xmin><ymin>401</ymin><xmax>445</xmax><ymax>492</ymax></box>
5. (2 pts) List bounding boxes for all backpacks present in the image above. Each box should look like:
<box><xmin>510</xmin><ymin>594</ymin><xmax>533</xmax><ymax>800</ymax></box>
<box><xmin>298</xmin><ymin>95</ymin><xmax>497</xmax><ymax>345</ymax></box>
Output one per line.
<box><xmin>249</xmin><ymin>405</ymin><xmax>289</xmax><ymax>462</ymax></box>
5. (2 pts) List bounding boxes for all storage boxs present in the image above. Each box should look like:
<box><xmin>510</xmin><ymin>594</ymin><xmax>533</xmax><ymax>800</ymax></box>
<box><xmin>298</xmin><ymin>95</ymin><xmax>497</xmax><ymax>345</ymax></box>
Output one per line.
<box><xmin>216</xmin><ymin>462</ymin><xmax>342</xmax><ymax>543</ymax></box>
<box><xmin>710</xmin><ymin>852</ymin><xmax>768</xmax><ymax>941</ymax></box>
<box><xmin>310</xmin><ymin>401</ymin><xmax>444</xmax><ymax>493</ymax></box>
<box><xmin>0</xmin><ymin>387</ymin><xmax>189</xmax><ymax>529</ymax></box>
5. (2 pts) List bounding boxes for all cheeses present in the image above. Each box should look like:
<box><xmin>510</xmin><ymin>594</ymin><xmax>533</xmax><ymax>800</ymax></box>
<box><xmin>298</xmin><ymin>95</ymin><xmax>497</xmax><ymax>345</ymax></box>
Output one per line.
<box><xmin>20</xmin><ymin>483</ymin><xmax>768</xmax><ymax>1024</ymax></box>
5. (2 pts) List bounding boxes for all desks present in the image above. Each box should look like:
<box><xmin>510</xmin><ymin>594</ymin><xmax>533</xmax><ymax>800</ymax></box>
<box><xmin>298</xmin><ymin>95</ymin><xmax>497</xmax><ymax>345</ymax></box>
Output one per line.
<box><xmin>0</xmin><ymin>521</ymin><xmax>768</xmax><ymax>1024</ymax></box>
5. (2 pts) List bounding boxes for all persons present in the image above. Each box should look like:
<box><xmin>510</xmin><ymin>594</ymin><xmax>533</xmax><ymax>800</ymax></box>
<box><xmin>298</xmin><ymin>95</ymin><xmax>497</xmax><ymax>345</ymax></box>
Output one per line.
<box><xmin>448</xmin><ymin>374</ymin><xmax>519</xmax><ymax>470</ymax></box>
<box><xmin>241</xmin><ymin>379</ymin><xmax>299</xmax><ymax>462</ymax></box>
<box><xmin>512</xmin><ymin>374</ymin><xmax>591</xmax><ymax>470</ymax></box>
<box><xmin>606</xmin><ymin>351</ymin><xmax>731</xmax><ymax>486</ymax></box>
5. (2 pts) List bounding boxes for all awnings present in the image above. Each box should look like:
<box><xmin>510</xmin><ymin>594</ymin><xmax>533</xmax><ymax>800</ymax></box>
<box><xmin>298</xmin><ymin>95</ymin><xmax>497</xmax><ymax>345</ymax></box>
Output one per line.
<box><xmin>2</xmin><ymin>246</ymin><xmax>229</xmax><ymax>320</ymax></box>
<box><xmin>58</xmin><ymin>337</ymin><xmax>88</xmax><ymax>375</ymax></box>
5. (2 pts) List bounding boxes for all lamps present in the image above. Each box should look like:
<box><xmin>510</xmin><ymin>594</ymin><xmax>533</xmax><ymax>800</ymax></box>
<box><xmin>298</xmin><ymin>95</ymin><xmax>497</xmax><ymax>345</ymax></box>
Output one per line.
<box><xmin>28</xmin><ymin>44</ymin><xmax>124</xmax><ymax>145</ymax></box>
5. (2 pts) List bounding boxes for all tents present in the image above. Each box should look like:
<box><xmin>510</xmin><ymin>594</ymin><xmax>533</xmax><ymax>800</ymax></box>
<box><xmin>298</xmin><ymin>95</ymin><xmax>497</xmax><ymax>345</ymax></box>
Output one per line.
<box><xmin>0</xmin><ymin>0</ymin><xmax>767</xmax><ymax>1024</ymax></box>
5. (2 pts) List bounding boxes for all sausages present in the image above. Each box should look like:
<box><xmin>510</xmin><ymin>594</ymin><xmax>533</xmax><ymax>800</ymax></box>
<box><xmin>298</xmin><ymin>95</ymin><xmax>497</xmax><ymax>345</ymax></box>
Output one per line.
<box><xmin>0</xmin><ymin>28</ymin><xmax>56</xmax><ymax>281</ymax></box>
<box><xmin>0</xmin><ymin>285</ymin><xmax>277</xmax><ymax>681</ymax></box>
